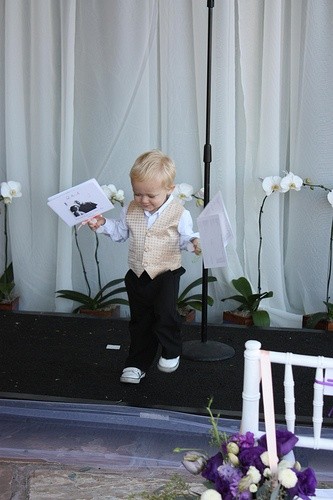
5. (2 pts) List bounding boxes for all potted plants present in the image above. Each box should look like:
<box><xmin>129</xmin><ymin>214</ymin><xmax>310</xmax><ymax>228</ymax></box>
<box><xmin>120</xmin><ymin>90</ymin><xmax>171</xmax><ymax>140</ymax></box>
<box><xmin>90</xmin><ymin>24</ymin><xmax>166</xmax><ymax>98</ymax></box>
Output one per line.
<box><xmin>223</xmin><ymin>275</ymin><xmax>274</xmax><ymax>325</ymax></box>
<box><xmin>302</xmin><ymin>301</ymin><xmax>333</xmax><ymax>330</ymax></box>
<box><xmin>172</xmin><ymin>275</ymin><xmax>217</xmax><ymax>321</ymax></box>
<box><xmin>58</xmin><ymin>278</ymin><xmax>130</xmax><ymax>317</ymax></box>
<box><xmin>0</xmin><ymin>262</ymin><xmax>21</xmax><ymax>311</ymax></box>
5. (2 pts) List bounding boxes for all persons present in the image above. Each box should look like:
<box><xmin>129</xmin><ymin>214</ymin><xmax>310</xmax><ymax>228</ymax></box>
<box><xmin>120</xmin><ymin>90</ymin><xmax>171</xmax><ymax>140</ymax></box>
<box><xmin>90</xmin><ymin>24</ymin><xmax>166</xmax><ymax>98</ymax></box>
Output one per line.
<box><xmin>88</xmin><ymin>149</ymin><xmax>202</xmax><ymax>383</ymax></box>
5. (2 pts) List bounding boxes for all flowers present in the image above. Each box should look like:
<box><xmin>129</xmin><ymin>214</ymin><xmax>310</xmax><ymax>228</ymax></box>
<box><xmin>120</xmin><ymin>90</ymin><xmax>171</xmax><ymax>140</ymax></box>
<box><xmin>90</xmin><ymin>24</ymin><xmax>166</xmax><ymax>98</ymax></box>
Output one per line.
<box><xmin>171</xmin><ymin>412</ymin><xmax>319</xmax><ymax>500</ymax></box>
<box><xmin>97</xmin><ymin>183</ymin><xmax>125</xmax><ymax>205</ymax></box>
<box><xmin>261</xmin><ymin>176</ymin><xmax>285</xmax><ymax>197</ymax></box>
<box><xmin>174</xmin><ymin>182</ymin><xmax>193</xmax><ymax>205</ymax></box>
<box><xmin>0</xmin><ymin>180</ymin><xmax>22</xmax><ymax>204</ymax></box>
<box><xmin>281</xmin><ymin>171</ymin><xmax>303</xmax><ymax>192</ymax></box>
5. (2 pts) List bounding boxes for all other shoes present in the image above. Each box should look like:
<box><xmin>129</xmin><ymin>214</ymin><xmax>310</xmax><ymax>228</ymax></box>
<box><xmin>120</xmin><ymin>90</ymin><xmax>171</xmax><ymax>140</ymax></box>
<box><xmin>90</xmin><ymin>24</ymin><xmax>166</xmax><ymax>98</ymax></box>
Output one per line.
<box><xmin>119</xmin><ymin>367</ymin><xmax>145</xmax><ymax>383</ymax></box>
<box><xmin>156</xmin><ymin>356</ymin><xmax>180</xmax><ymax>372</ymax></box>
